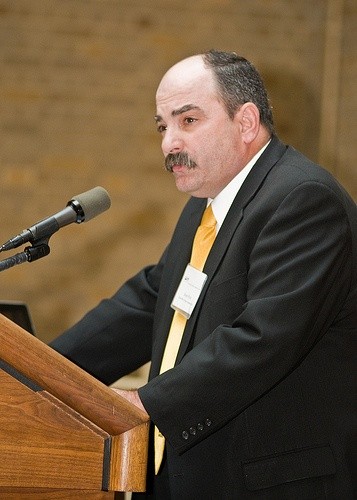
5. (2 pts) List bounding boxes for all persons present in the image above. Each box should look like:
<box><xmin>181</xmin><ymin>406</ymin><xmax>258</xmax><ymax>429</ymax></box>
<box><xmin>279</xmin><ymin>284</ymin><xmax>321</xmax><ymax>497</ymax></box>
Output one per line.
<box><xmin>49</xmin><ymin>49</ymin><xmax>357</xmax><ymax>500</ymax></box>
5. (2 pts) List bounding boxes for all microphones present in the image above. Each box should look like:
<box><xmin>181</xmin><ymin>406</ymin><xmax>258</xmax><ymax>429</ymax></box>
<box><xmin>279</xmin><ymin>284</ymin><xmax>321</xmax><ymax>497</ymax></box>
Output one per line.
<box><xmin>2</xmin><ymin>186</ymin><xmax>111</xmax><ymax>251</ymax></box>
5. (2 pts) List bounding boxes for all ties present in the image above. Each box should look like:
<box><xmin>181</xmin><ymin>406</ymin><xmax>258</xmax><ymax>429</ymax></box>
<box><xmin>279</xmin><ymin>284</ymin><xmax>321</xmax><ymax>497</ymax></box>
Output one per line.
<box><xmin>154</xmin><ymin>202</ymin><xmax>220</xmax><ymax>476</ymax></box>
